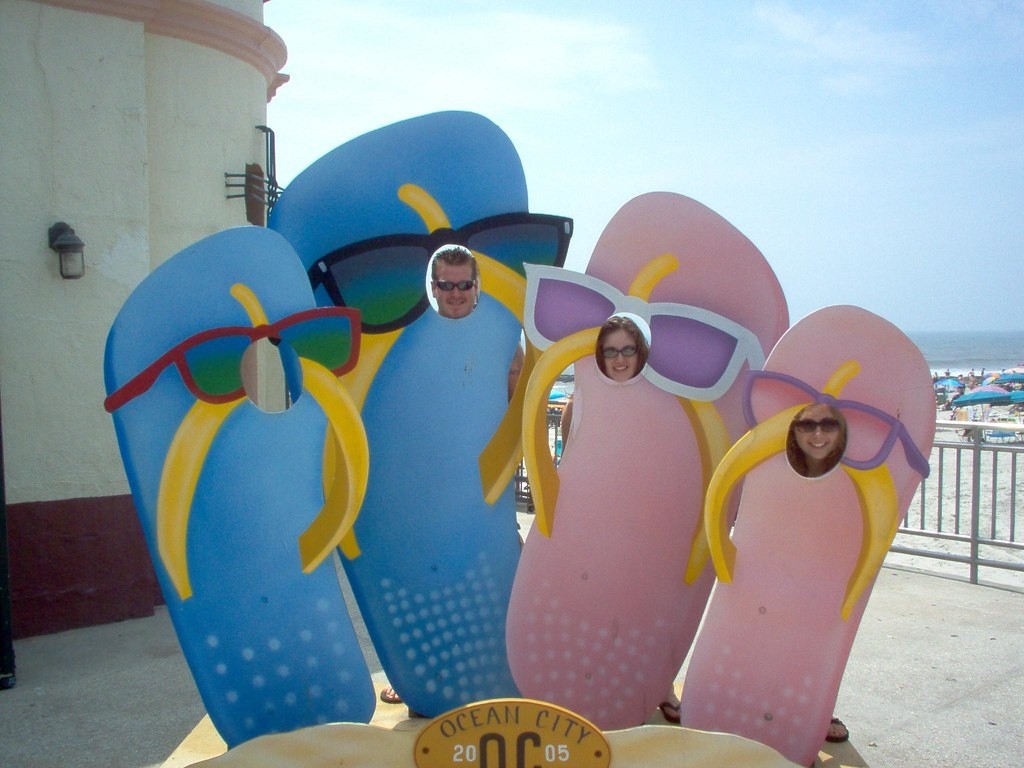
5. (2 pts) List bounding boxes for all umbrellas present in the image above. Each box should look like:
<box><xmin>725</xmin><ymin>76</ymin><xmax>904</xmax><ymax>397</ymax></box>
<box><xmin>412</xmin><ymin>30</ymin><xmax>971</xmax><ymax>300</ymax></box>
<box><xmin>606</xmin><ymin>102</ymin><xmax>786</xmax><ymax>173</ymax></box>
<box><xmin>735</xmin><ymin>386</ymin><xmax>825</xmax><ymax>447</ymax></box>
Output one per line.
<box><xmin>935</xmin><ymin>368</ymin><xmax>1024</xmax><ymax>423</ymax></box>
<box><xmin>549</xmin><ymin>390</ymin><xmax>567</xmax><ymax>399</ymax></box>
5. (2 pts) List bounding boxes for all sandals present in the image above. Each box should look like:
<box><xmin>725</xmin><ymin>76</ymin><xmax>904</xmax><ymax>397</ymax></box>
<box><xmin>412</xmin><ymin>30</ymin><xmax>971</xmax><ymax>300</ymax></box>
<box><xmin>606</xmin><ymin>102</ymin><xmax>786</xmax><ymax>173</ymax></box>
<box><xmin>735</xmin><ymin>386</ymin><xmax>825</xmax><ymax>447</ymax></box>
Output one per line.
<box><xmin>658</xmin><ymin>699</ymin><xmax>681</xmax><ymax>723</ymax></box>
<box><xmin>826</xmin><ymin>717</ymin><xmax>849</xmax><ymax>744</ymax></box>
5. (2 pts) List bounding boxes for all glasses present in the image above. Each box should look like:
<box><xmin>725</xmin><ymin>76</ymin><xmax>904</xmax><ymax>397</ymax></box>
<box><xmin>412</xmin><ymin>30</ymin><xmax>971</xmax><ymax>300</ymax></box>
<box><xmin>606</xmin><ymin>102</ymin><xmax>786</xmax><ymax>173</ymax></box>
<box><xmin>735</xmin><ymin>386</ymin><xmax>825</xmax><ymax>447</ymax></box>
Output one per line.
<box><xmin>602</xmin><ymin>345</ymin><xmax>638</xmax><ymax>358</ymax></box>
<box><xmin>792</xmin><ymin>418</ymin><xmax>840</xmax><ymax>433</ymax></box>
<box><xmin>435</xmin><ymin>279</ymin><xmax>474</xmax><ymax>292</ymax></box>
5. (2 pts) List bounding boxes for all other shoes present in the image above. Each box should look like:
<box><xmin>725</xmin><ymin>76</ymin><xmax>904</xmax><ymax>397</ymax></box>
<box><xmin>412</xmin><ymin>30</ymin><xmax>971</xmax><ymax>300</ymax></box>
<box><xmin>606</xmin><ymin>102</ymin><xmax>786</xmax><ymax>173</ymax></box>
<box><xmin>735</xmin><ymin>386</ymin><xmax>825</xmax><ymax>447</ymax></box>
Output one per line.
<box><xmin>381</xmin><ymin>687</ymin><xmax>402</xmax><ymax>703</ymax></box>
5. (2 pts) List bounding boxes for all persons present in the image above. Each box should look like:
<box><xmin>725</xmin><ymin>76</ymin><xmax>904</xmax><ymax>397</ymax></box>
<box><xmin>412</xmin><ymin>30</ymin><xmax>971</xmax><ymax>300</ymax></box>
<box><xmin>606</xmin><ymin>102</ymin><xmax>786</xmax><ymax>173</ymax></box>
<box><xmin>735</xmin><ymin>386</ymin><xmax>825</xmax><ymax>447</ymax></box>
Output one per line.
<box><xmin>546</xmin><ymin>407</ymin><xmax>564</xmax><ymax>428</ymax></box>
<box><xmin>430</xmin><ymin>249</ymin><xmax>525</xmax><ymax>405</ymax></box>
<box><xmin>932</xmin><ymin>368</ymin><xmax>1024</xmax><ymax>442</ymax></box>
<box><xmin>562</xmin><ymin>315</ymin><xmax>650</xmax><ymax>457</ymax></box>
<box><xmin>787</xmin><ymin>402</ymin><xmax>848</xmax><ymax>478</ymax></box>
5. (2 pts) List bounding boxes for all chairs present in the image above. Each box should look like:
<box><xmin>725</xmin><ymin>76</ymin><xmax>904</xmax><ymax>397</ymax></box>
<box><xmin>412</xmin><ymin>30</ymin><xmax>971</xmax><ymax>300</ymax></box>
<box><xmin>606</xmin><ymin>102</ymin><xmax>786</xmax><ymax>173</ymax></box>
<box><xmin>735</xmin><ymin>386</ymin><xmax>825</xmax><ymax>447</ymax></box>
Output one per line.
<box><xmin>954</xmin><ymin>430</ymin><xmax>969</xmax><ymax>442</ymax></box>
<box><xmin>986</xmin><ymin>430</ymin><xmax>1015</xmax><ymax>444</ymax></box>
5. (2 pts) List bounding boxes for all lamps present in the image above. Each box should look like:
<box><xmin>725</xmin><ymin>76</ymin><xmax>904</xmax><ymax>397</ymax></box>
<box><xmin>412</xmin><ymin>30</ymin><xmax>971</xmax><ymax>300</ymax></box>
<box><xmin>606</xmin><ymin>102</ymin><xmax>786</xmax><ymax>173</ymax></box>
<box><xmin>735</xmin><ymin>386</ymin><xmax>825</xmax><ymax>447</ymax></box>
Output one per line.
<box><xmin>48</xmin><ymin>222</ymin><xmax>86</xmax><ymax>279</ymax></box>
